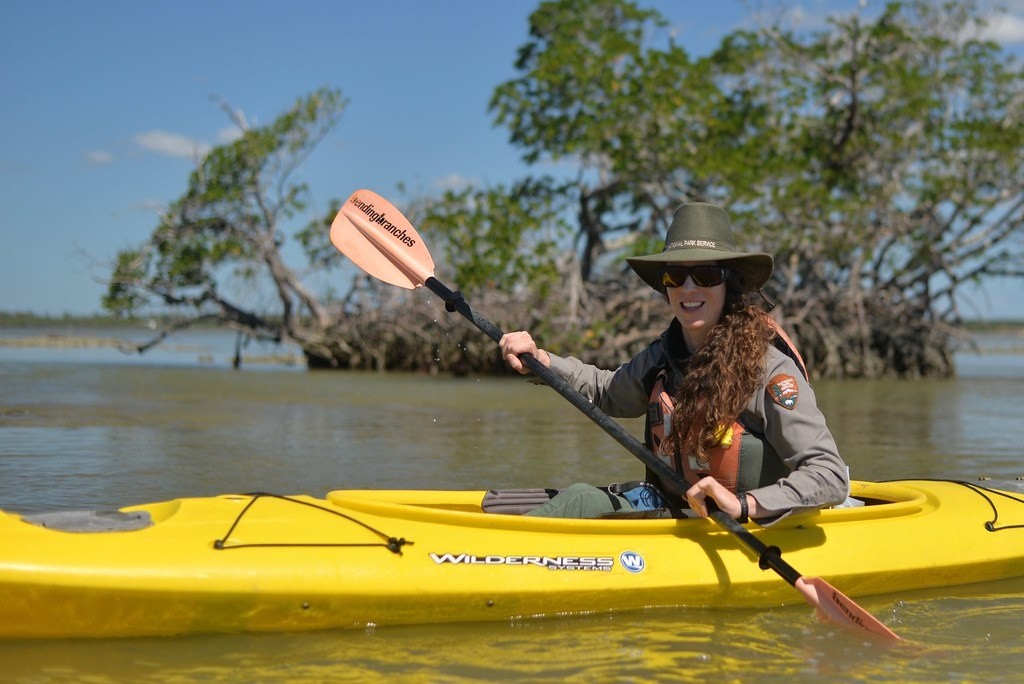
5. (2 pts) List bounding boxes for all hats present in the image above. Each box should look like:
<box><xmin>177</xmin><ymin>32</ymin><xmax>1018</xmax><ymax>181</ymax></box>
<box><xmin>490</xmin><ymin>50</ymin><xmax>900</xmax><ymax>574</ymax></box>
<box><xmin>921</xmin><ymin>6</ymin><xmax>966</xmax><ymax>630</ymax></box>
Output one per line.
<box><xmin>625</xmin><ymin>203</ymin><xmax>774</xmax><ymax>307</ymax></box>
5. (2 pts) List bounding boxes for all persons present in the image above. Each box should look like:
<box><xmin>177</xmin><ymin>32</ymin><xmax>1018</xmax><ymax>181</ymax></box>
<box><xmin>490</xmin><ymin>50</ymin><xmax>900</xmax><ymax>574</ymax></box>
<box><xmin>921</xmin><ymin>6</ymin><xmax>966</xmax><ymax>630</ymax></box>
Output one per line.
<box><xmin>496</xmin><ymin>201</ymin><xmax>849</xmax><ymax>528</ymax></box>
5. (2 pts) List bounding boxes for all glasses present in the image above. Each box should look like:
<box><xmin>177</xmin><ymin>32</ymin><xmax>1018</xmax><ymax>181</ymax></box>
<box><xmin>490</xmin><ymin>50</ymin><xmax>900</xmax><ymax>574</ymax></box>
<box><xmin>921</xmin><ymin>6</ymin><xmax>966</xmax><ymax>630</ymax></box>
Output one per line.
<box><xmin>658</xmin><ymin>264</ymin><xmax>731</xmax><ymax>287</ymax></box>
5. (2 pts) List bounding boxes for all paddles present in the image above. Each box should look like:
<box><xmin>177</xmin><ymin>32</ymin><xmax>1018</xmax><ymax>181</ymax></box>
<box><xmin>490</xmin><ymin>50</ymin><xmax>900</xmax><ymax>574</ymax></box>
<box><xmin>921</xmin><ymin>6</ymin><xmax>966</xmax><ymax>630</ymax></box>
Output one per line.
<box><xmin>325</xmin><ymin>187</ymin><xmax>902</xmax><ymax>641</ymax></box>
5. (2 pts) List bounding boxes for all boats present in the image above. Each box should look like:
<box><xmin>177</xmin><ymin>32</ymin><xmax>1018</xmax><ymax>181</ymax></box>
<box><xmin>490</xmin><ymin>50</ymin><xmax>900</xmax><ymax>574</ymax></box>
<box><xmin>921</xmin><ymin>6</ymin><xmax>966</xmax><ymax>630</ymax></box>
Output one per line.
<box><xmin>0</xmin><ymin>476</ymin><xmax>1023</xmax><ymax>642</ymax></box>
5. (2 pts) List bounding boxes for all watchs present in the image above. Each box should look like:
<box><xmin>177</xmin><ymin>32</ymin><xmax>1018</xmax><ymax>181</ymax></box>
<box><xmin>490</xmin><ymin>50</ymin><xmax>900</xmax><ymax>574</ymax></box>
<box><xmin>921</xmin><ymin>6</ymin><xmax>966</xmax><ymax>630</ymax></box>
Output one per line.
<box><xmin>735</xmin><ymin>492</ymin><xmax>749</xmax><ymax>523</ymax></box>
